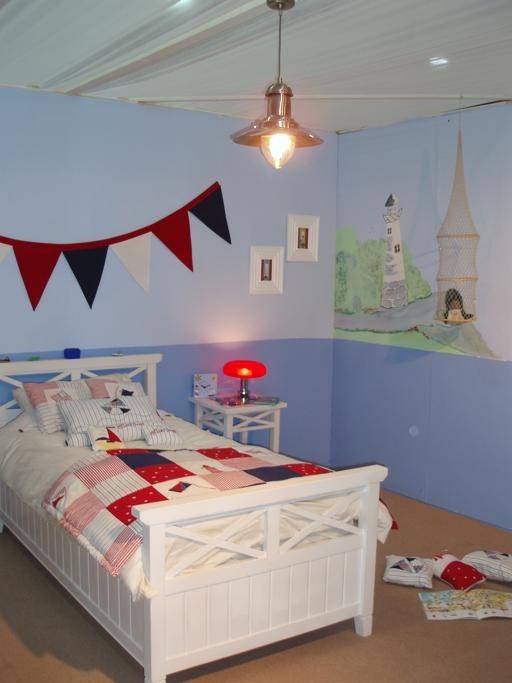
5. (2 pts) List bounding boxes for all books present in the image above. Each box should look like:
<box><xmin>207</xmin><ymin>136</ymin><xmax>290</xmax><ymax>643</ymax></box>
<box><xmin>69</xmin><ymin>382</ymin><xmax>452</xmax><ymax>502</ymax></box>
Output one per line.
<box><xmin>417</xmin><ymin>589</ymin><xmax>511</xmax><ymax>620</ymax></box>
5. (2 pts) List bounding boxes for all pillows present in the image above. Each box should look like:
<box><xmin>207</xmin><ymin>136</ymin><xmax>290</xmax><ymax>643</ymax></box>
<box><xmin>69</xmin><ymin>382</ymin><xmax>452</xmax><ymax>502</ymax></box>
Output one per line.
<box><xmin>383</xmin><ymin>549</ymin><xmax>512</xmax><ymax>592</ymax></box>
<box><xmin>12</xmin><ymin>372</ymin><xmax>189</xmax><ymax>455</ymax></box>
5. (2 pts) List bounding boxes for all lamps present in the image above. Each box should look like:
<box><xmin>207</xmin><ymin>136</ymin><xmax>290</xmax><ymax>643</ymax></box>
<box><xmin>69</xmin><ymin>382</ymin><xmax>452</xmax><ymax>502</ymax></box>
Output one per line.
<box><xmin>222</xmin><ymin>358</ymin><xmax>269</xmax><ymax>405</ymax></box>
<box><xmin>231</xmin><ymin>0</ymin><xmax>325</xmax><ymax>170</ymax></box>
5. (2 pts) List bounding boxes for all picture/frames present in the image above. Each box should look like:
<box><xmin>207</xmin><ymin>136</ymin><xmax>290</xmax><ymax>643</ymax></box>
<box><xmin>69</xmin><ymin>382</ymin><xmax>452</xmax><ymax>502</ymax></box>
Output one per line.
<box><xmin>250</xmin><ymin>213</ymin><xmax>320</xmax><ymax>296</ymax></box>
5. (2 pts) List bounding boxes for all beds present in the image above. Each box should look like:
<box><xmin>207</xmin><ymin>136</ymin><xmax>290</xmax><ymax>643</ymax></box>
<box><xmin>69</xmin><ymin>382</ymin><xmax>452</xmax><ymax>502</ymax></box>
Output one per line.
<box><xmin>0</xmin><ymin>353</ymin><xmax>396</xmax><ymax>682</ymax></box>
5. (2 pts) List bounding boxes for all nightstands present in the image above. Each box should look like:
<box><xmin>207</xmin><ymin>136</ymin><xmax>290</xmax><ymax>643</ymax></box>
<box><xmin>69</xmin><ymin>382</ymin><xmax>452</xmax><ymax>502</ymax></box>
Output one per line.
<box><xmin>190</xmin><ymin>391</ymin><xmax>286</xmax><ymax>453</ymax></box>
